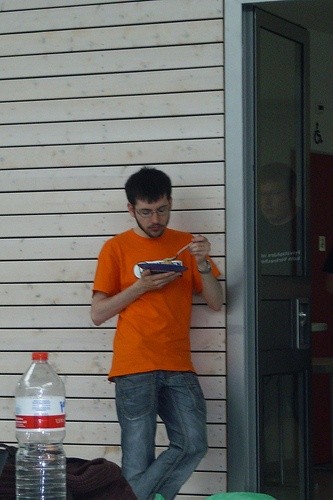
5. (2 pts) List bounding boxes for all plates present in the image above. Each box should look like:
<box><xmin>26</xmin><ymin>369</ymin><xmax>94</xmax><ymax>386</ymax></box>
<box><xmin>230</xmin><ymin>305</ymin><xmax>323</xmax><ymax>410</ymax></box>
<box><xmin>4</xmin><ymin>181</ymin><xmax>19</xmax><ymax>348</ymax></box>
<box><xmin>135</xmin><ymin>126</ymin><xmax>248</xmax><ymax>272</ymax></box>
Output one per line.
<box><xmin>137</xmin><ymin>263</ymin><xmax>188</xmax><ymax>275</ymax></box>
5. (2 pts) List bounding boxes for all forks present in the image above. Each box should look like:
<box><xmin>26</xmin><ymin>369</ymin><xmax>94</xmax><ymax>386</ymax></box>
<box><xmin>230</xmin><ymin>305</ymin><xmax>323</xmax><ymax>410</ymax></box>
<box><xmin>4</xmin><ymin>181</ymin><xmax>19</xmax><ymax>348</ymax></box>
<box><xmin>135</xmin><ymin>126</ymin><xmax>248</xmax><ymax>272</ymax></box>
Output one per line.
<box><xmin>163</xmin><ymin>234</ymin><xmax>202</xmax><ymax>261</ymax></box>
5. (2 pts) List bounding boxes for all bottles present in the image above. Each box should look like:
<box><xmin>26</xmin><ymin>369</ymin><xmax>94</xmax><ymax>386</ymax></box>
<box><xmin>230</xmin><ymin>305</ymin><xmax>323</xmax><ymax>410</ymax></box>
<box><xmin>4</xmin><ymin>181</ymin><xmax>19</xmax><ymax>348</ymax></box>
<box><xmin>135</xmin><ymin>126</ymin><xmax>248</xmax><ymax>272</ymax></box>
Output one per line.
<box><xmin>14</xmin><ymin>351</ymin><xmax>66</xmax><ymax>500</ymax></box>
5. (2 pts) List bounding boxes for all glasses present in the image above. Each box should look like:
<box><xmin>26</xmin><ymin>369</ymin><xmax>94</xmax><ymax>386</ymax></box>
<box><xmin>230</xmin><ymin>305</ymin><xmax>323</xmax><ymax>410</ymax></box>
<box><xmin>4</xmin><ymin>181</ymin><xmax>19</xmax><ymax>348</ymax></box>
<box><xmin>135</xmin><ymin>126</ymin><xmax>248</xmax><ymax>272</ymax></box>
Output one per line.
<box><xmin>132</xmin><ymin>200</ymin><xmax>171</xmax><ymax>217</ymax></box>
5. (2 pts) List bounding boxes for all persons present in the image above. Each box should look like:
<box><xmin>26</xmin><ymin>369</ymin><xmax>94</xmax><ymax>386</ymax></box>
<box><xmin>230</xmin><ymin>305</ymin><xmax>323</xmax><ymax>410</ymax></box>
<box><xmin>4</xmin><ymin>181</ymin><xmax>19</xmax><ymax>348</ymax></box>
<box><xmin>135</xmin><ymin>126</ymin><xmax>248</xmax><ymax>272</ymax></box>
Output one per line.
<box><xmin>90</xmin><ymin>166</ymin><xmax>224</xmax><ymax>500</ymax></box>
<box><xmin>256</xmin><ymin>161</ymin><xmax>297</xmax><ymax>276</ymax></box>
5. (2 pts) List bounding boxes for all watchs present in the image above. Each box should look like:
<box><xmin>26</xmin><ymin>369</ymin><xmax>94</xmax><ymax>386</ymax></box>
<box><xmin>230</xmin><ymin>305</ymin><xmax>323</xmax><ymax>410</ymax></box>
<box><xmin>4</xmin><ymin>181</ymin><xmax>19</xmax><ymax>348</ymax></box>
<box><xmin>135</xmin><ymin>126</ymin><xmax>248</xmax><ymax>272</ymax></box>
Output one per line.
<box><xmin>196</xmin><ymin>258</ymin><xmax>212</xmax><ymax>274</ymax></box>
